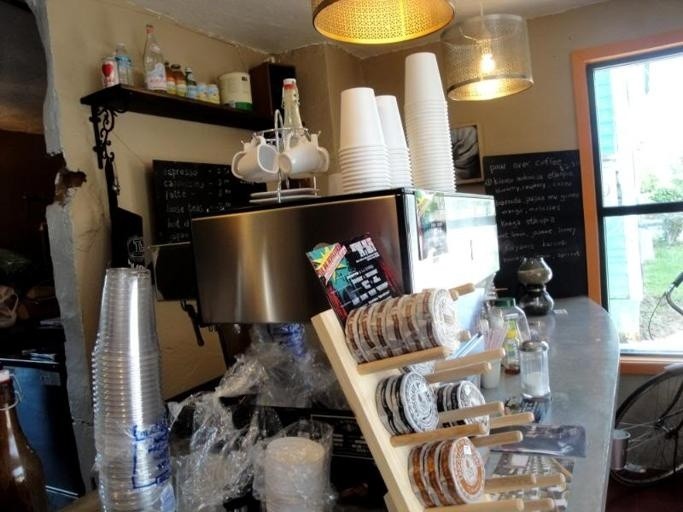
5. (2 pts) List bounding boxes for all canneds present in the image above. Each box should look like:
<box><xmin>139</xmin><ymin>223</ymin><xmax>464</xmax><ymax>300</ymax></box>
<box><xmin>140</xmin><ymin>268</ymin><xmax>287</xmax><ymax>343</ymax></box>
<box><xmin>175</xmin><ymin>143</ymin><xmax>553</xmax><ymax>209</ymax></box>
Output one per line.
<box><xmin>99</xmin><ymin>56</ymin><xmax>118</xmax><ymax>90</ymax></box>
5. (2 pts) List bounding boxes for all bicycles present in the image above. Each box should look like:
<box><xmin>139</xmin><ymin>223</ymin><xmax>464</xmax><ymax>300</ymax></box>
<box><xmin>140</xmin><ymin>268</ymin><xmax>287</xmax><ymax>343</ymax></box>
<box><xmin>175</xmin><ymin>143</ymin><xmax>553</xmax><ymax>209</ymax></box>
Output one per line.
<box><xmin>611</xmin><ymin>266</ymin><xmax>683</xmax><ymax>491</ymax></box>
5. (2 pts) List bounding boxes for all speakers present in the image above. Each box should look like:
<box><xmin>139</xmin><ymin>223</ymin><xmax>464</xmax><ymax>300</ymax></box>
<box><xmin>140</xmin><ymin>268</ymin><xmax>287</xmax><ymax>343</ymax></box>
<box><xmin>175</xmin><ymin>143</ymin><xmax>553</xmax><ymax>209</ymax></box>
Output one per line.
<box><xmin>248</xmin><ymin>62</ymin><xmax>296</xmax><ymax>119</ymax></box>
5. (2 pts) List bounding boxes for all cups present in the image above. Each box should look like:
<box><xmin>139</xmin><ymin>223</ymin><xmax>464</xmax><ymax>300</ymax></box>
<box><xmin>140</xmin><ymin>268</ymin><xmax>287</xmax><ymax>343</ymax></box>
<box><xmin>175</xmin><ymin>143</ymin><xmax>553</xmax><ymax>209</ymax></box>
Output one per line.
<box><xmin>262</xmin><ymin>436</ymin><xmax>327</xmax><ymax>512</ymax></box>
<box><xmin>337</xmin><ymin>50</ymin><xmax>457</xmax><ymax>195</ymax></box>
<box><xmin>91</xmin><ymin>264</ymin><xmax>179</xmax><ymax>512</ymax></box>
<box><xmin>279</xmin><ymin>131</ymin><xmax>330</xmax><ymax>180</ymax></box>
<box><xmin>231</xmin><ymin>134</ymin><xmax>279</xmax><ymax>183</ymax></box>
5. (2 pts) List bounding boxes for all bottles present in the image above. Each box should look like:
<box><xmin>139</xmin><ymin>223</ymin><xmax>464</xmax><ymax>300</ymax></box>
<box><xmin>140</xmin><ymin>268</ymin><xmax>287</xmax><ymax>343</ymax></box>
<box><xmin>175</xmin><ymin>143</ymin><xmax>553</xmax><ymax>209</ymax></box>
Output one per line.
<box><xmin>115</xmin><ymin>23</ymin><xmax>197</xmax><ymax>100</ymax></box>
<box><xmin>500</xmin><ymin>312</ymin><xmax>524</xmax><ymax>374</ymax></box>
<box><xmin>519</xmin><ymin>340</ymin><xmax>552</xmax><ymax>401</ymax></box>
<box><xmin>516</xmin><ymin>254</ymin><xmax>554</xmax><ymax>317</ymax></box>
<box><xmin>266</xmin><ymin>77</ymin><xmax>318</xmax><ymax>196</ymax></box>
<box><xmin>490</xmin><ymin>298</ymin><xmax>531</xmax><ymax>343</ymax></box>
<box><xmin>0</xmin><ymin>370</ymin><xmax>49</xmax><ymax>512</ymax></box>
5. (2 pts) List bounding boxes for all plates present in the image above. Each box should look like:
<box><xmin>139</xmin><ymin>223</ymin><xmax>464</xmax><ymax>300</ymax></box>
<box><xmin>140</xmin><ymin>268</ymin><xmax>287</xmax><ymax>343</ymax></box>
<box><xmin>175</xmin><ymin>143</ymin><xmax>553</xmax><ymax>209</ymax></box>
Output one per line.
<box><xmin>248</xmin><ymin>187</ymin><xmax>321</xmax><ymax>204</ymax></box>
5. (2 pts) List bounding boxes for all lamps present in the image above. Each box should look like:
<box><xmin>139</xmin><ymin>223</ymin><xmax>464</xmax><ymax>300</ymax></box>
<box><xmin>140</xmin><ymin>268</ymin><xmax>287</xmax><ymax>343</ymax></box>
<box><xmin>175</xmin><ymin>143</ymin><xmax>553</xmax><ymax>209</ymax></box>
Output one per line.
<box><xmin>437</xmin><ymin>0</ymin><xmax>534</xmax><ymax>104</ymax></box>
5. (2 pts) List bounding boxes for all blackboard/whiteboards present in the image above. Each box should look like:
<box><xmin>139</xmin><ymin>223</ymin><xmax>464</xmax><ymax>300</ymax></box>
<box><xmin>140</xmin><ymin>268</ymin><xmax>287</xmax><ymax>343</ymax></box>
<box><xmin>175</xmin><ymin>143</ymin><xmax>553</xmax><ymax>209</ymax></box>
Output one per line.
<box><xmin>483</xmin><ymin>150</ymin><xmax>589</xmax><ymax>300</ymax></box>
<box><xmin>152</xmin><ymin>159</ymin><xmax>310</xmax><ymax>246</ymax></box>
